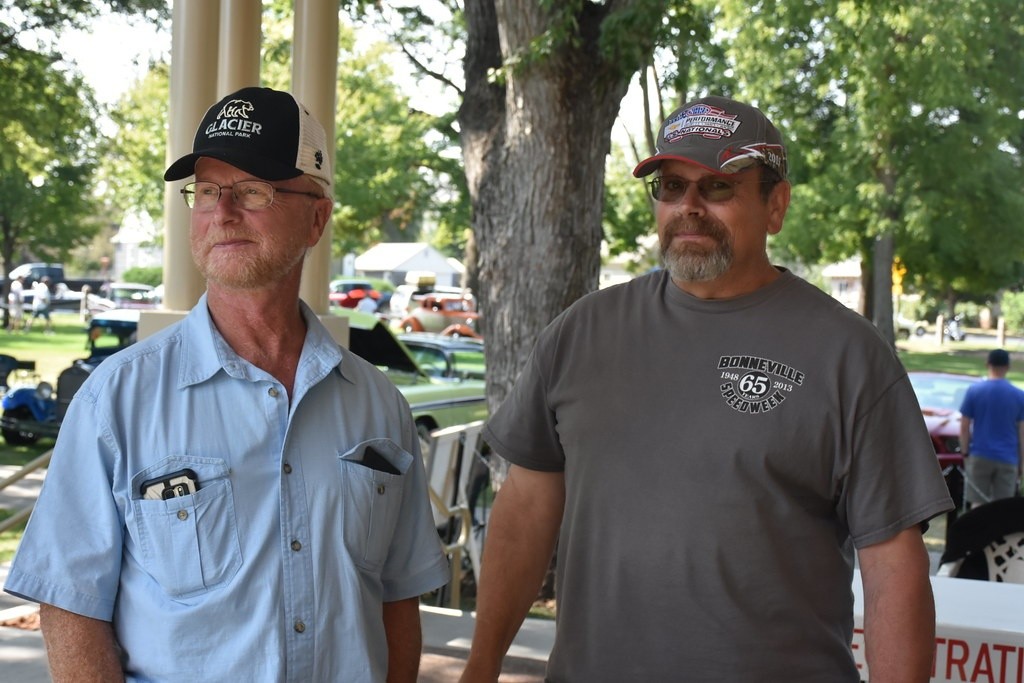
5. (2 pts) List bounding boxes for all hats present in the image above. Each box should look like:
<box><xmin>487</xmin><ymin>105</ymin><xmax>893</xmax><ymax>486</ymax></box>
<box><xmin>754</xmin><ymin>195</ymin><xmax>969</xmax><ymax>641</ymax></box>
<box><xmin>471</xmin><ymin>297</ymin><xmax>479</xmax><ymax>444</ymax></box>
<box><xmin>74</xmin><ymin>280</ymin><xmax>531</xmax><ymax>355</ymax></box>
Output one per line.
<box><xmin>164</xmin><ymin>86</ymin><xmax>331</xmax><ymax>186</ymax></box>
<box><xmin>632</xmin><ymin>96</ymin><xmax>787</xmax><ymax>181</ymax></box>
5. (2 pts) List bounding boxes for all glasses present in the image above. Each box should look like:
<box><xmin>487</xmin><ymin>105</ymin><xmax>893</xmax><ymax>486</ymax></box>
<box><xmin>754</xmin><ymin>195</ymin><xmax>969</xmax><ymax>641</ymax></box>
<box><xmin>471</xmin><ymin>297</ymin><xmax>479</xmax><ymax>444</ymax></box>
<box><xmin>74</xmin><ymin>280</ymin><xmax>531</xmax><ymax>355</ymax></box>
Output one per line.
<box><xmin>181</xmin><ymin>180</ymin><xmax>323</xmax><ymax>212</ymax></box>
<box><xmin>646</xmin><ymin>173</ymin><xmax>778</xmax><ymax>202</ymax></box>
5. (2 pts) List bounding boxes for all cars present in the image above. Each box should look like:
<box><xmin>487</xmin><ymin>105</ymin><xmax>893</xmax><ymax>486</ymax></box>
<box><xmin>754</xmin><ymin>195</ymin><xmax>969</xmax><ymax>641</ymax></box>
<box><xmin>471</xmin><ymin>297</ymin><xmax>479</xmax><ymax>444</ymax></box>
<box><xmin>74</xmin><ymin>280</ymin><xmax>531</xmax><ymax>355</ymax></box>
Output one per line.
<box><xmin>96</xmin><ymin>282</ymin><xmax>158</xmax><ymax>311</ymax></box>
<box><xmin>904</xmin><ymin>373</ymin><xmax>986</xmax><ymax>507</ymax></box>
<box><xmin>329</xmin><ymin>281</ymin><xmax>377</xmax><ymax>309</ymax></box>
<box><xmin>327</xmin><ymin>309</ymin><xmax>492</xmax><ymax>474</ymax></box>
<box><xmin>894</xmin><ymin>312</ymin><xmax>916</xmax><ymax>342</ymax></box>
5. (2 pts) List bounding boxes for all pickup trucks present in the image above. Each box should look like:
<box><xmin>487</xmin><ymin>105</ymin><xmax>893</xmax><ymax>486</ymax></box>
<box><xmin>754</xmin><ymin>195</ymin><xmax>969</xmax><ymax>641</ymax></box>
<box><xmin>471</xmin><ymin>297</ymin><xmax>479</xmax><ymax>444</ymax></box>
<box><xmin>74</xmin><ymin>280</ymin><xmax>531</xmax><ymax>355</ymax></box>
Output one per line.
<box><xmin>0</xmin><ymin>262</ymin><xmax>113</xmax><ymax>306</ymax></box>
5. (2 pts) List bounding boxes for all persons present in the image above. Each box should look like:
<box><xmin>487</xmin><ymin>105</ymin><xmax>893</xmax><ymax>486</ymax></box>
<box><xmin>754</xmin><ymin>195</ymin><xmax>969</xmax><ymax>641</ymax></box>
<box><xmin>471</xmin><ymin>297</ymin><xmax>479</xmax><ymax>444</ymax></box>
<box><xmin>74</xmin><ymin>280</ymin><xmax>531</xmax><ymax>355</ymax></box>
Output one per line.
<box><xmin>959</xmin><ymin>349</ymin><xmax>1024</xmax><ymax>508</ymax></box>
<box><xmin>461</xmin><ymin>94</ymin><xmax>956</xmax><ymax>683</ymax></box>
<box><xmin>3</xmin><ymin>86</ymin><xmax>456</xmax><ymax>683</ymax></box>
<box><xmin>353</xmin><ymin>286</ymin><xmax>392</xmax><ymax>314</ymax></box>
<box><xmin>97</xmin><ymin>277</ymin><xmax>114</xmax><ymax>302</ymax></box>
<box><xmin>7</xmin><ymin>276</ymin><xmax>56</xmax><ymax>335</ymax></box>
